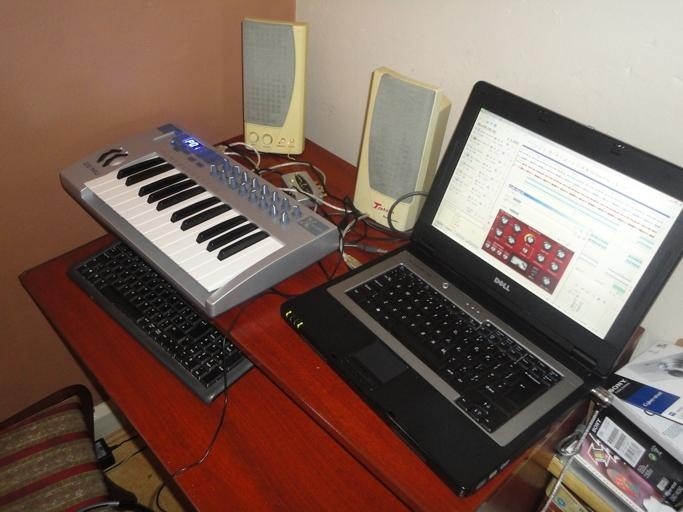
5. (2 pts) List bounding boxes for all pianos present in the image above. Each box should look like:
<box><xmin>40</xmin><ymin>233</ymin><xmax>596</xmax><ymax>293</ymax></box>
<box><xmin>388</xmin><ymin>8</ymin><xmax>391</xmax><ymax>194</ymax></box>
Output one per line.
<box><xmin>58</xmin><ymin>120</ymin><xmax>342</xmax><ymax>320</ymax></box>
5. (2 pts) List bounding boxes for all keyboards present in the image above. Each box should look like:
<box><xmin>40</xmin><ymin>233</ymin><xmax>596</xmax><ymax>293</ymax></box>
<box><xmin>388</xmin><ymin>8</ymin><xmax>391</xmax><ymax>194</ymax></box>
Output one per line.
<box><xmin>67</xmin><ymin>239</ymin><xmax>254</xmax><ymax>405</ymax></box>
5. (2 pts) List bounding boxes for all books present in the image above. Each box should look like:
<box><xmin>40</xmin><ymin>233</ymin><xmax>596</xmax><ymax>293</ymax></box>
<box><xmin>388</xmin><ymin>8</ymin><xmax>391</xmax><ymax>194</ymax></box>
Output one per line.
<box><xmin>544</xmin><ymin>339</ymin><xmax>683</xmax><ymax>512</ymax></box>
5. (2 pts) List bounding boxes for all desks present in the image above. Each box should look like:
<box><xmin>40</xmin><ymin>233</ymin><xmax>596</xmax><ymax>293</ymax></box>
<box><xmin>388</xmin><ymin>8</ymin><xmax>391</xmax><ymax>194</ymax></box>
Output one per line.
<box><xmin>17</xmin><ymin>139</ymin><xmax>592</xmax><ymax>511</ymax></box>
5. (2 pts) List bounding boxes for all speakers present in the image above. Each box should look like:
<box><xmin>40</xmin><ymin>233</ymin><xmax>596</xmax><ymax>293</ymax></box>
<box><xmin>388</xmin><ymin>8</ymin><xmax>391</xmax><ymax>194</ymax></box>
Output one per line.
<box><xmin>351</xmin><ymin>68</ymin><xmax>452</xmax><ymax>232</ymax></box>
<box><xmin>240</xmin><ymin>18</ymin><xmax>307</xmax><ymax>154</ymax></box>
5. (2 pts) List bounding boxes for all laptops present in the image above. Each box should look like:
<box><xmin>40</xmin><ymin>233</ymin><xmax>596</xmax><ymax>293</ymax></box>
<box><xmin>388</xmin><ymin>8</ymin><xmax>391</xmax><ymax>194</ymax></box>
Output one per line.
<box><xmin>280</xmin><ymin>82</ymin><xmax>683</xmax><ymax>497</ymax></box>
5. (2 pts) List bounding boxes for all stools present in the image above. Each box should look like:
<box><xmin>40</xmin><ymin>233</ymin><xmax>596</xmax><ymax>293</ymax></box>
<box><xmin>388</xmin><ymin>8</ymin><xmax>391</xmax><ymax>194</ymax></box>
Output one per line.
<box><xmin>0</xmin><ymin>383</ymin><xmax>139</xmax><ymax>512</ymax></box>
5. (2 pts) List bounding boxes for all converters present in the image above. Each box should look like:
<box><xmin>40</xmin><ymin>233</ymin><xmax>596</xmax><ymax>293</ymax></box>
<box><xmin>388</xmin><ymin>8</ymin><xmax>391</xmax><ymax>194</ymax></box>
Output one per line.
<box><xmin>96</xmin><ymin>436</ymin><xmax>115</xmax><ymax>467</ymax></box>
